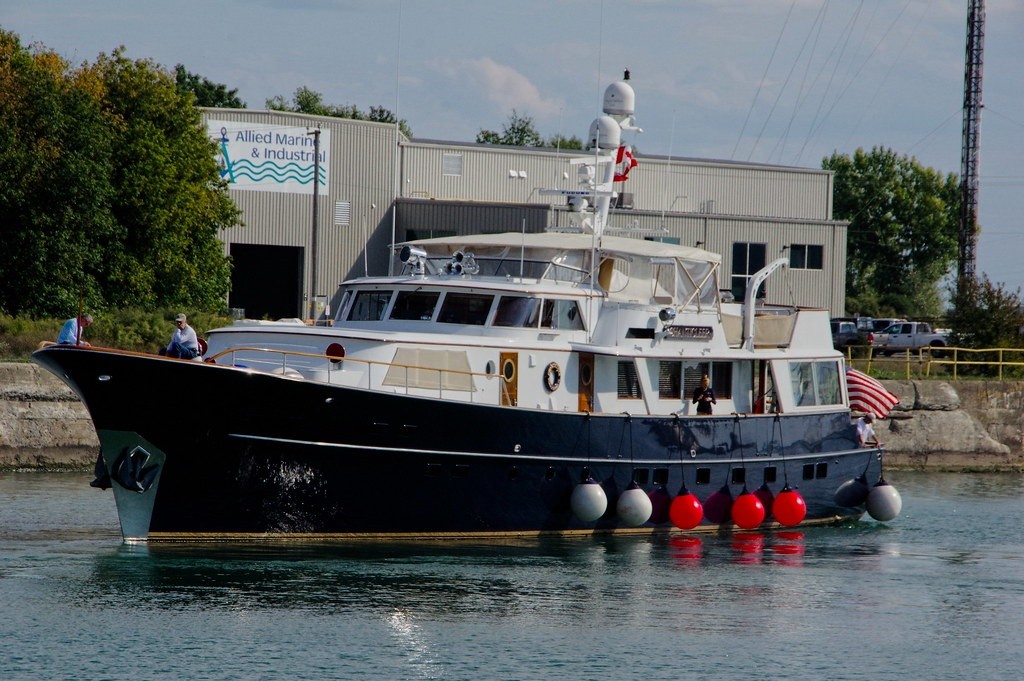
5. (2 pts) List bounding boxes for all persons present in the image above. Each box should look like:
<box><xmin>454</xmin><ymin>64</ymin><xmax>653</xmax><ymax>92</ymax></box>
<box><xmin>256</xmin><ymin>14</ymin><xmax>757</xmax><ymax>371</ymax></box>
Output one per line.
<box><xmin>58</xmin><ymin>313</ymin><xmax>93</xmax><ymax>348</ymax></box>
<box><xmin>165</xmin><ymin>313</ymin><xmax>202</xmax><ymax>363</ymax></box>
<box><xmin>691</xmin><ymin>374</ymin><xmax>716</xmax><ymax>416</ymax></box>
<box><xmin>856</xmin><ymin>411</ymin><xmax>880</xmax><ymax>449</ymax></box>
<box><xmin>866</xmin><ymin>330</ymin><xmax>874</xmax><ymax>345</ymax></box>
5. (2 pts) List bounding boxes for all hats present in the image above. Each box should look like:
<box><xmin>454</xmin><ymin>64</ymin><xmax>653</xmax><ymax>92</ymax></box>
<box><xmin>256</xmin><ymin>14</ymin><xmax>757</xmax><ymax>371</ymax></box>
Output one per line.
<box><xmin>175</xmin><ymin>313</ymin><xmax>186</xmax><ymax>321</ymax></box>
<box><xmin>868</xmin><ymin>412</ymin><xmax>876</xmax><ymax>424</ymax></box>
<box><xmin>701</xmin><ymin>375</ymin><xmax>709</xmax><ymax>380</ymax></box>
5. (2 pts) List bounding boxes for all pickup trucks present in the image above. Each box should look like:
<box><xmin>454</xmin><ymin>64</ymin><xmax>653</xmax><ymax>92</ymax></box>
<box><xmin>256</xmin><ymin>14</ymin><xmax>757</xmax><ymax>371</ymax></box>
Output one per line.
<box><xmin>874</xmin><ymin>322</ymin><xmax>962</xmax><ymax>359</ymax></box>
<box><xmin>833</xmin><ymin>315</ymin><xmax>905</xmax><ymax>337</ymax></box>
<box><xmin>829</xmin><ymin>320</ymin><xmax>889</xmax><ymax>358</ymax></box>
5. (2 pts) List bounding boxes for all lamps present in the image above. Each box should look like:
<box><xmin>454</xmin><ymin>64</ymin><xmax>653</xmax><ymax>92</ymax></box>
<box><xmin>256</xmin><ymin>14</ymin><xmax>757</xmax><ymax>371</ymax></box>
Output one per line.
<box><xmin>400</xmin><ymin>246</ymin><xmax>428</xmax><ymax>280</ymax></box>
<box><xmin>442</xmin><ymin>250</ymin><xmax>479</xmax><ymax>277</ymax></box>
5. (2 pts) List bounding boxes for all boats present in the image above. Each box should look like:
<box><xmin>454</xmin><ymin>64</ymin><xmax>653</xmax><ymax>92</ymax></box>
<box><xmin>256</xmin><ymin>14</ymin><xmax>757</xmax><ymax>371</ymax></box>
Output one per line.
<box><xmin>29</xmin><ymin>77</ymin><xmax>890</xmax><ymax>556</ymax></box>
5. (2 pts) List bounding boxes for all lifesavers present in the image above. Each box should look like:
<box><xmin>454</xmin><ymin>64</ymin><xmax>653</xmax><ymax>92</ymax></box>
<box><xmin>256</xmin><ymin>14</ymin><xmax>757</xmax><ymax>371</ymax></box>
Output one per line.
<box><xmin>544</xmin><ymin>362</ymin><xmax>561</xmax><ymax>391</ymax></box>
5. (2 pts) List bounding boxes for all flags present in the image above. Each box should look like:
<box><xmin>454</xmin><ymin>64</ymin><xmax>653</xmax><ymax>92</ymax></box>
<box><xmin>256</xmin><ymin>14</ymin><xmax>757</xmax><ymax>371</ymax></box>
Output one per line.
<box><xmin>613</xmin><ymin>143</ymin><xmax>638</xmax><ymax>184</ymax></box>
<box><xmin>845</xmin><ymin>363</ymin><xmax>900</xmax><ymax>419</ymax></box>
